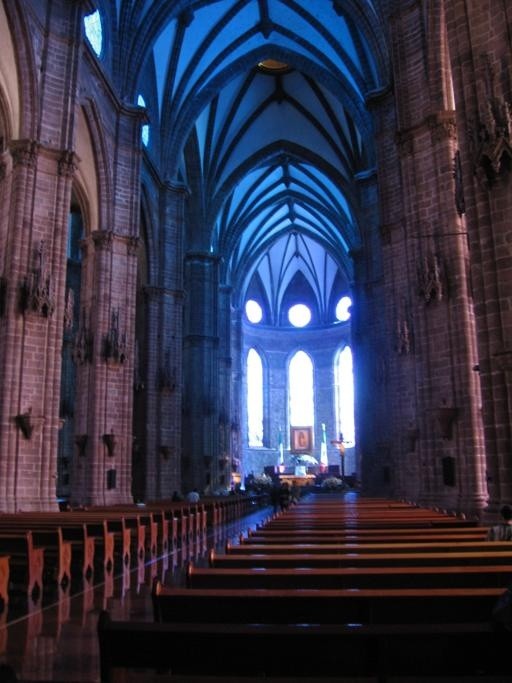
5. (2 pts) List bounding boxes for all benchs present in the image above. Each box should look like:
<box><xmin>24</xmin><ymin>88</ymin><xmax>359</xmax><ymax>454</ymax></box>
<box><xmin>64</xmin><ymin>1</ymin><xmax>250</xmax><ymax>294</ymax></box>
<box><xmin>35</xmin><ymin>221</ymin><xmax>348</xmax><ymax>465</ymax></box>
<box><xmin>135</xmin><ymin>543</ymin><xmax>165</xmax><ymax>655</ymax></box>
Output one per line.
<box><xmin>3</xmin><ymin>496</ymin><xmax>510</xmax><ymax>682</ymax></box>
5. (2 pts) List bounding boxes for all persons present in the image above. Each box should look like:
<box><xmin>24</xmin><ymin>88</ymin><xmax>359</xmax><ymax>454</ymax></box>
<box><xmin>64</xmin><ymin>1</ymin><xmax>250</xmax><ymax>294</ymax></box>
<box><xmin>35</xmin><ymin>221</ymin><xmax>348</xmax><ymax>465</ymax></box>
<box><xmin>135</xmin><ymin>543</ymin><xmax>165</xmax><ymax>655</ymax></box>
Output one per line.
<box><xmin>271</xmin><ymin>474</ymin><xmax>282</xmax><ymax>515</ymax></box>
<box><xmin>172</xmin><ymin>490</ymin><xmax>181</xmax><ymax>501</ymax></box>
<box><xmin>279</xmin><ymin>484</ymin><xmax>291</xmax><ymax>511</ymax></box>
<box><xmin>186</xmin><ymin>488</ymin><xmax>202</xmax><ymax>503</ymax></box>
